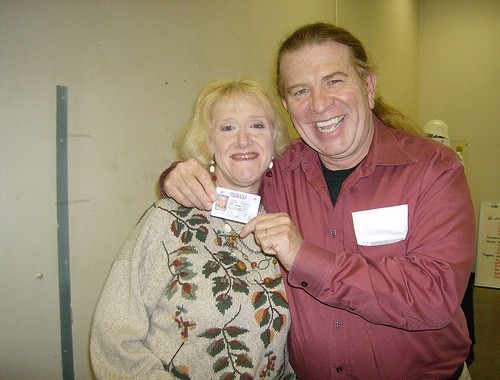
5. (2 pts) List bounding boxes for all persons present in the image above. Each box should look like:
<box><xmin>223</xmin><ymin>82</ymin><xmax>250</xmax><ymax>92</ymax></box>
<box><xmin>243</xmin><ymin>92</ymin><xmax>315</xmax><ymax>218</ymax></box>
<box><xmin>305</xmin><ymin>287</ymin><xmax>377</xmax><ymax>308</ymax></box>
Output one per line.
<box><xmin>158</xmin><ymin>22</ymin><xmax>476</xmax><ymax>380</ymax></box>
<box><xmin>90</xmin><ymin>77</ymin><xmax>298</xmax><ymax>380</ymax></box>
